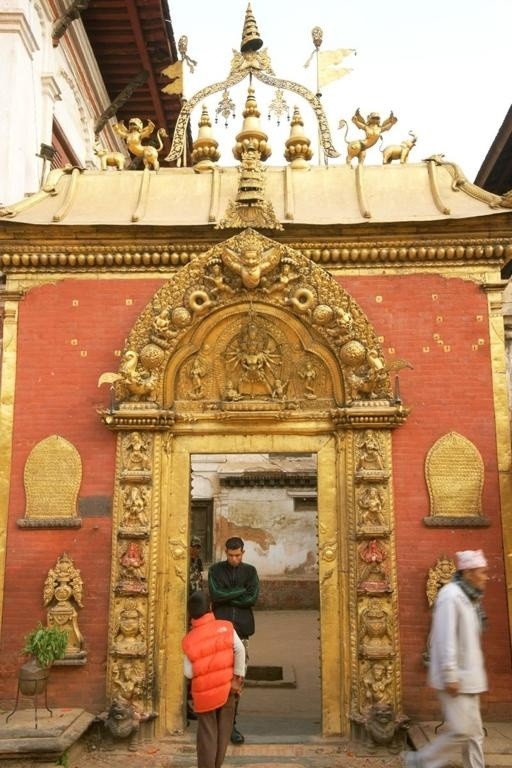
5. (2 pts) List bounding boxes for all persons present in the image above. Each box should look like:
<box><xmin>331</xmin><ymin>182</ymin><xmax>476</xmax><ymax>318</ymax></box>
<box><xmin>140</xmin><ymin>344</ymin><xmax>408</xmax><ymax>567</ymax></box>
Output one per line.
<box><xmin>182</xmin><ymin>242</ymin><xmax>319</xmax><ymax>408</ymax></box>
<box><xmin>110</xmin><ymin>431</ymin><xmax>154</xmax><ymax>714</ymax></box>
<box><xmin>111</xmin><ymin>116</ymin><xmax>156</xmax><ymax>154</ymax></box>
<box><xmin>181</xmin><ymin>590</ymin><xmax>249</xmax><ymax>768</ymax></box>
<box><xmin>206</xmin><ymin>536</ymin><xmax>261</xmax><ymax>746</ymax></box>
<box><xmin>397</xmin><ymin>548</ymin><xmax>488</xmax><ymax>766</ymax></box>
<box><xmin>349</xmin><ymin>423</ymin><xmax>396</xmax><ymax>726</ymax></box>
<box><xmin>186</xmin><ymin>535</ymin><xmax>204</xmax><ymax>722</ymax></box>
<box><xmin>350</xmin><ymin>104</ymin><xmax>397</xmax><ymax>148</ymax></box>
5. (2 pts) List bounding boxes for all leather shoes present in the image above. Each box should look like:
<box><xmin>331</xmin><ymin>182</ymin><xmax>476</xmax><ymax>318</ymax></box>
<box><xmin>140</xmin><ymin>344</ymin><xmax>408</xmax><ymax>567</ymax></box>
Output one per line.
<box><xmin>231</xmin><ymin>727</ymin><xmax>244</xmax><ymax>744</ymax></box>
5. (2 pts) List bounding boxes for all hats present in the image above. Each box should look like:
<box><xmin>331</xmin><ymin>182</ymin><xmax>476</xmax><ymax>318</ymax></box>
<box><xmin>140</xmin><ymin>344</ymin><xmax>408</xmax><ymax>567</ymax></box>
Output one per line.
<box><xmin>456</xmin><ymin>549</ymin><xmax>487</xmax><ymax>570</ymax></box>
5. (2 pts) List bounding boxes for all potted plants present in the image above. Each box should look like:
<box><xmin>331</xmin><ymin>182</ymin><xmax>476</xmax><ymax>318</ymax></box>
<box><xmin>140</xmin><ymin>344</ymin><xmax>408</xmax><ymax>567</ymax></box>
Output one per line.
<box><xmin>19</xmin><ymin>621</ymin><xmax>68</xmax><ymax>694</ymax></box>
